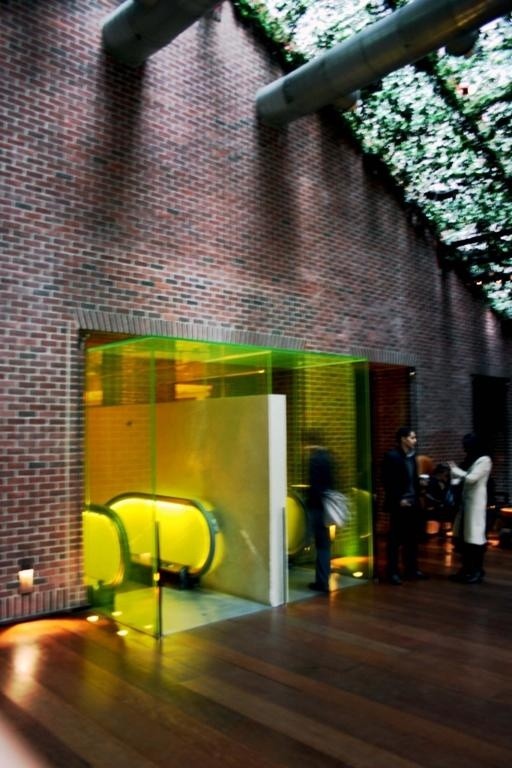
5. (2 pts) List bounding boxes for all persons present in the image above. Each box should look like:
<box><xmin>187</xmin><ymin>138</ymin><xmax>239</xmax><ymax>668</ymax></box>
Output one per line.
<box><xmin>384</xmin><ymin>428</ymin><xmax>431</xmax><ymax>584</ymax></box>
<box><xmin>421</xmin><ymin>432</ymin><xmax>493</xmax><ymax>584</ymax></box>
<box><xmin>301</xmin><ymin>428</ymin><xmax>336</xmax><ymax>593</ymax></box>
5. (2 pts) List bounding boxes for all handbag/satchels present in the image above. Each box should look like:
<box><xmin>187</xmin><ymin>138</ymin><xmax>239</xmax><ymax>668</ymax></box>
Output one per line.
<box><xmin>322</xmin><ymin>489</ymin><xmax>350</xmax><ymax>527</ymax></box>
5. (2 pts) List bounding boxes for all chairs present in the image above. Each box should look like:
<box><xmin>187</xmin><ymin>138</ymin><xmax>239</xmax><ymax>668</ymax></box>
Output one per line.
<box><xmin>414</xmin><ymin>453</ymin><xmax>456</xmax><ymax>543</ymax></box>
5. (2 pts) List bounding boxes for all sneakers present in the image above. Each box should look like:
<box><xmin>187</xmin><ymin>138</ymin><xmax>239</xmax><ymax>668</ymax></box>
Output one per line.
<box><xmin>308</xmin><ymin>582</ymin><xmax>330</xmax><ymax>592</ymax></box>
<box><xmin>389</xmin><ymin>568</ymin><xmax>487</xmax><ymax>585</ymax></box>
